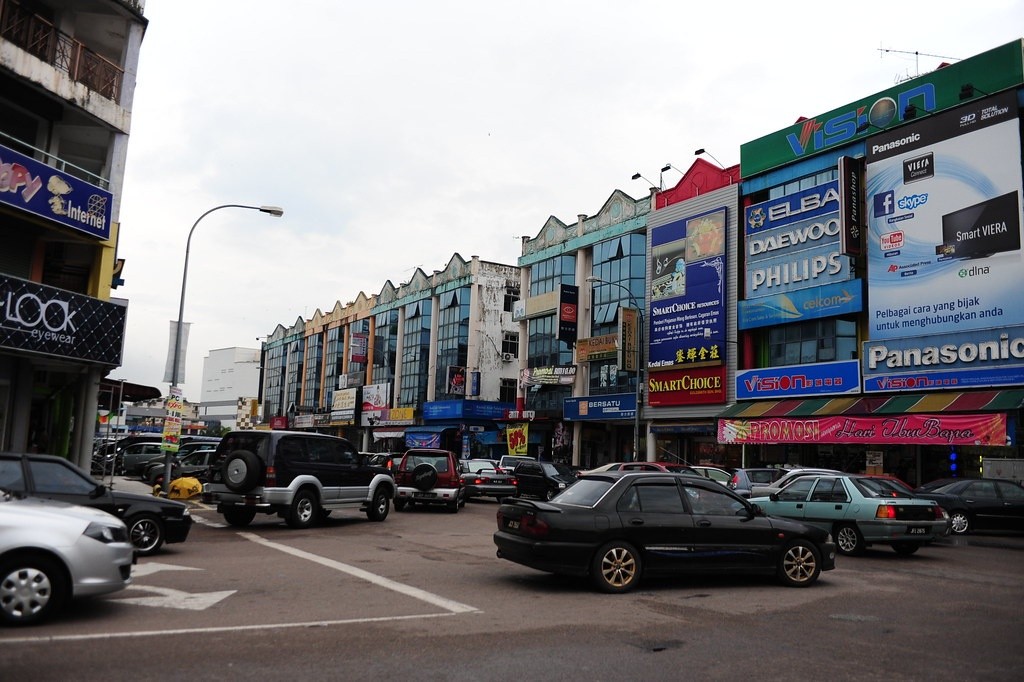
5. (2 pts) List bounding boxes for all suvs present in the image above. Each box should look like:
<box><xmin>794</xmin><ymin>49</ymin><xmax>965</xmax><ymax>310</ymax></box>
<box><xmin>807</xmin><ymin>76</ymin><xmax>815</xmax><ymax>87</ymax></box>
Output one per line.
<box><xmin>200</xmin><ymin>430</ymin><xmax>396</xmax><ymax>528</ymax></box>
<box><xmin>393</xmin><ymin>449</ymin><xmax>467</xmax><ymax>513</ymax></box>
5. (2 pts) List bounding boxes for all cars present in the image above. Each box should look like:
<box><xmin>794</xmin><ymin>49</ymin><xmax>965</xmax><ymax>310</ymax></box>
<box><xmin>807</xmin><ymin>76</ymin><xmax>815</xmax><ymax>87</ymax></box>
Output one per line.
<box><xmin>917</xmin><ymin>477</ymin><xmax>1024</xmax><ymax>536</ymax></box>
<box><xmin>577</xmin><ymin>461</ymin><xmax>914</xmax><ymax>508</ymax></box>
<box><xmin>458</xmin><ymin>460</ymin><xmax>519</xmax><ymax>499</ymax></box>
<box><xmin>0</xmin><ymin>488</ymin><xmax>136</xmax><ymax>622</ymax></box>
<box><xmin>499</xmin><ymin>456</ymin><xmax>537</xmax><ymax>476</ymax></box>
<box><xmin>515</xmin><ymin>461</ymin><xmax>577</xmax><ymax>501</ymax></box>
<box><xmin>494</xmin><ymin>470</ymin><xmax>837</xmax><ymax>594</ymax></box>
<box><xmin>367</xmin><ymin>452</ymin><xmax>422</xmax><ymax>477</ymax></box>
<box><xmin>91</xmin><ymin>430</ymin><xmax>222</xmax><ymax>489</ymax></box>
<box><xmin>733</xmin><ymin>474</ymin><xmax>948</xmax><ymax>557</ymax></box>
<box><xmin>0</xmin><ymin>452</ymin><xmax>193</xmax><ymax>556</ymax></box>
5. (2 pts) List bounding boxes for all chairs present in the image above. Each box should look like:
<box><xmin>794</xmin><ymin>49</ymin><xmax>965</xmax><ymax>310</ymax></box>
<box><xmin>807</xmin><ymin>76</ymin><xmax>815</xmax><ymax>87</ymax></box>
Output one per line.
<box><xmin>435</xmin><ymin>460</ymin><xmax>447</xmax><ymax>467</ymax></box>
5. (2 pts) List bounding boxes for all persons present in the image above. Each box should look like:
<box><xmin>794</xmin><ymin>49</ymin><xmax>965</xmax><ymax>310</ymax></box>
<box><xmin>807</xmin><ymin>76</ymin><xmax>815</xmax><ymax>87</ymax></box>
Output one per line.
<box><xmin>153</xmin><ymin>474</ymin><xmax>163</xmax><ymax>498</ymax></box>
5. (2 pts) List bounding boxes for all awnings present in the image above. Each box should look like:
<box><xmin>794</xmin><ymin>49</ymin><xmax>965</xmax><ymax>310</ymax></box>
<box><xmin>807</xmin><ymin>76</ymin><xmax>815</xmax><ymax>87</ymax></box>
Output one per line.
<box><xmin>373</xmin><ymin>427</ymin><xmax>406</xmax><ymax>435</ymax></box>
<box><xmin>404</xmin><ymin>426</ymin><xmax>460</xmax><ymax>432</ymax></box>
<box><xmin>717</xmin><ymin>388</ymin><xmax>1023</xmax><ymax>418</ymax></box>
<box><xmin>474</xmin><ymin>430</ymin><xmax>542</xmax><ymax>445</ymax></box>
<box><xmin>99</xmin><ymin>376</ymin><xmax>162</xmax><ymax>410</ymax></box>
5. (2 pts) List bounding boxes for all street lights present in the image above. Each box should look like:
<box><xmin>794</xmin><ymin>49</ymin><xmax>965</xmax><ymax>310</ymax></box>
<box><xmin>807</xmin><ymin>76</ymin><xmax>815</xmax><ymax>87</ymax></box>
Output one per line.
<box><xmin>255</xmin><ymin>366</ymin><xmax>310</xmax><ymax>419</ymax></box>
<box><xmin>350</xmin><ymin>344</ymin><xmax>388</xmax><ymax>409</ymax></box>
<box><xmin>585</xmin><ymin>276</ymin><xmax>642</xmax><ymax>463</ymax></box>
<box><xmin>163</xmin><ymin>203</ymin><xmax>284</xmax><ymax>497</ymax></box>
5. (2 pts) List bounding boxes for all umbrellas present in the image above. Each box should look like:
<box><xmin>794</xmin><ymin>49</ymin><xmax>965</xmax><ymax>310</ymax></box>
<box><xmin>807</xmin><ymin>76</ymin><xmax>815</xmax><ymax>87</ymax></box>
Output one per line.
<box><xmin>167</xmin><ymin>477</ymin><xmax>202</xmax><ymax>499</ymax></box>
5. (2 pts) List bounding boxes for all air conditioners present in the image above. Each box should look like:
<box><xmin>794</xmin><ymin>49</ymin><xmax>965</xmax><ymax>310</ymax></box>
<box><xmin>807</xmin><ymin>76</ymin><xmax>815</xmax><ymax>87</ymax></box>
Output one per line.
<box><xmin>502</xmin><ymin>353</ymin><xmax>514</xmax><ymax>361</ymax></box>
